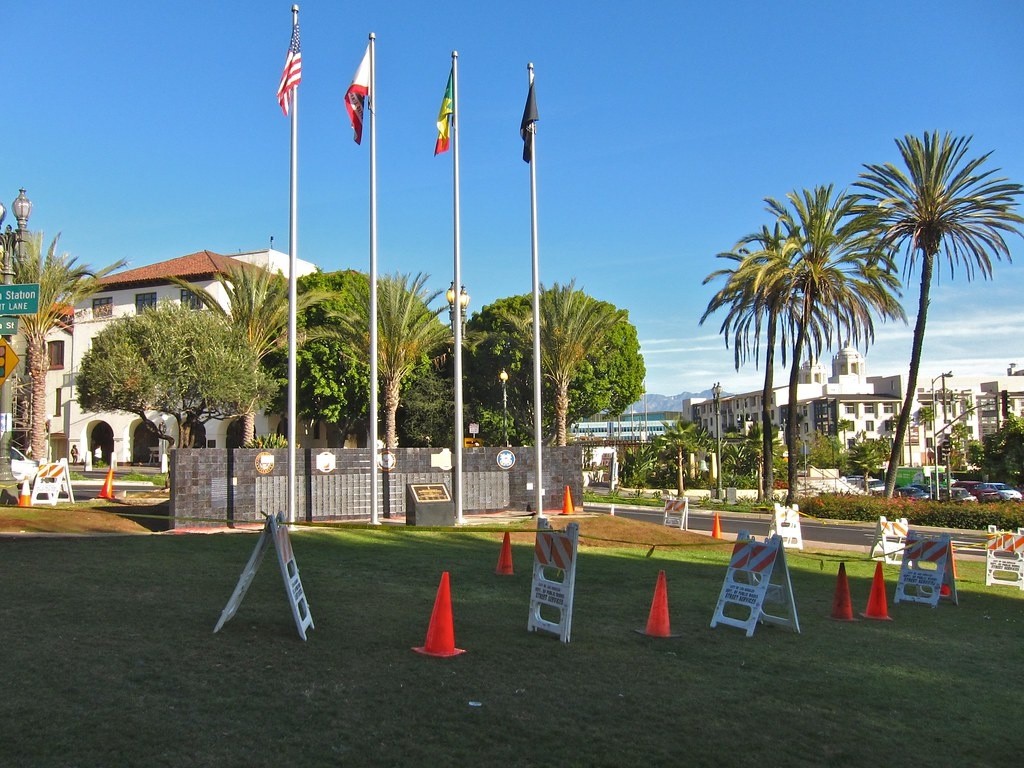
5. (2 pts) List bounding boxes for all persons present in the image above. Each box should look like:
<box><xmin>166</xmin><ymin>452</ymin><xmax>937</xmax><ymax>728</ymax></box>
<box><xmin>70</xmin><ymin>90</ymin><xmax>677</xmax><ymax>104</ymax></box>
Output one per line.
<box><xmin>94</xmin><ymin>445</ymin><xmax>102</xmax><ymax>466</ymax></box>
<box><xmin>71</xmin><ymin>445</ymin><xmax>78</xmax><ymax>464</ymax></box>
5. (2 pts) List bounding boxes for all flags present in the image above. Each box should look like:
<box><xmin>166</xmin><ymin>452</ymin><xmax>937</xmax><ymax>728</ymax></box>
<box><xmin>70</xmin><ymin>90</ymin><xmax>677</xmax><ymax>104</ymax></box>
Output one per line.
<box><xmin>344</xmin><ymin>42</ymin><xmax>370</xmax><ymax>145</ymax></box>
<box><xmin>277</xmin><ymin>23</ymin><xmax>301</xmax><ymax>116</ymax></box>
<box><xmin>520</xmin><ymin>79</ymin><xmax>539</xmax><ymax>163</ymax></box>
<box><xmin>435</xmin><ymin>69</ymin><xmax>454</xmax><ymax>157</ymax></box>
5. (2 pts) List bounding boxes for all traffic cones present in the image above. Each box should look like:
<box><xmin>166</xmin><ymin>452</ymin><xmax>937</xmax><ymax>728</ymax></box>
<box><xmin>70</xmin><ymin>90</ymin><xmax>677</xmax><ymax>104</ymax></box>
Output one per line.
<box><xmin>16</xmin><ymin>476</ymin><xmax>33</xmax><ymax>507</ymax></box>
<box><xmin>858</xmin><ymin>562</ymin><xmax>893</xmax><ymax>621</ymax></box>
<box><xmin>634</xmin><ymin>570</ymin><xmax>682</xmax><ymax>638</ymax></box>
<box><xmin>711</xmin><ymin>513</ymin><xmax>723</xmax><ymax>539</ymax></box>
<box><xmin>492</xmin><ymin>531</ymin><xmax>516</xmax><ymax>576</ymax></box>
<box><xmin>97</xmin><ymin>468</ymin><xmax>116</xmax><ymax>499</ymax></box>
<box><xmin>557</xmin><ymin>486</ymin><xmax>577</xmax><ymax>515</ymax></box>
<box><xmin>410</xmin><ymin>572</ymin><xmax>467</xmax><ymax>656</ymax></box>
<box><xmin>825</xmin><ymin>563</ymin><xmax>859</xmax><ymax>621</ymax></box>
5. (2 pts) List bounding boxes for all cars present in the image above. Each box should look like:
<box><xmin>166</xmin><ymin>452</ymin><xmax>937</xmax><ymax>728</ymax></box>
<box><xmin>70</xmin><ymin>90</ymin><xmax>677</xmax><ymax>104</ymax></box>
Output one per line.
<box><xmin>939</xmin><ymin>487</ymin><xmax>979</xmax><ymax>503</ymax></box>
<box><xmin>951</xmin><ymin>480</ymin><xmax>1006</xmax><ymax>503</ymax></box>
<box><xmin>984</xmin><ymin>483</ymin><xmax>1022</xmax><ymax>503</ymax></box>
<box><xmin>910</xmin><ymin>485</ymin><xmax>936</xmax><ymax>500</ymax></box>
<box><xmin>858</xmin><ymin>479</ymin><xmax>885</xmax><ymax>496</ymax></box>
<box><xmin>893</xmin><ymin>487</ymin><xmax>929</xmax><ymax>500</ymax></box>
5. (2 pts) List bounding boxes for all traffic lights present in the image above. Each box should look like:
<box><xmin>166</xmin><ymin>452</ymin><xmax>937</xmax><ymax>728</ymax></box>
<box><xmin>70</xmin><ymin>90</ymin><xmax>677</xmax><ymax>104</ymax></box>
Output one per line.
<box><xmin>1001</xmin><ymin>390</ymin><xmax>1011</xmax><ymax>416</ymax></box>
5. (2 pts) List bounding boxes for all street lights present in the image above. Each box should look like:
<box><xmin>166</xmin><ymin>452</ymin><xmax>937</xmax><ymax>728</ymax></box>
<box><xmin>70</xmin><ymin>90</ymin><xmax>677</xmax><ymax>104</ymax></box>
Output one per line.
<box><xmin>711</xmin><ymin>382</ymin><xmax>724</xmax><ymax>500</ymax></box>
<box><xmin>500</xmin><ymin>368</ymin><xmax>509</xmax><ymax>447</ymax></box>
<box><xmin>932</xmin><ymin>374</ymin><xmax>953</xmax><ymax>501</ymax></box>
<box><xmin>447</xmin><ymin>282</ymin><xmax>471</xmax><ymax>526</ymax></box>
